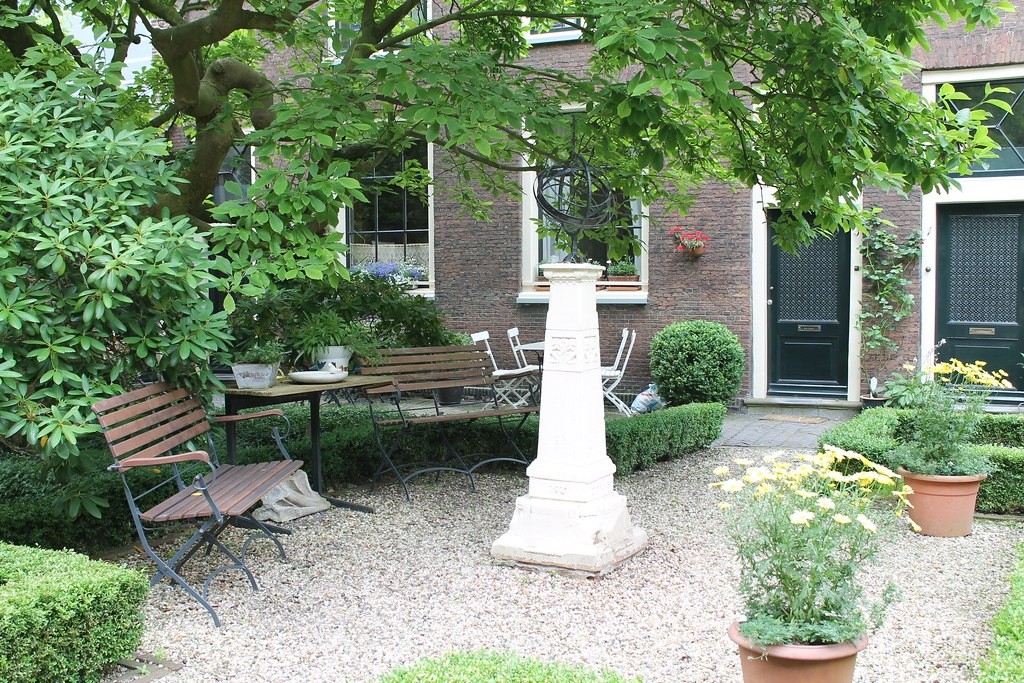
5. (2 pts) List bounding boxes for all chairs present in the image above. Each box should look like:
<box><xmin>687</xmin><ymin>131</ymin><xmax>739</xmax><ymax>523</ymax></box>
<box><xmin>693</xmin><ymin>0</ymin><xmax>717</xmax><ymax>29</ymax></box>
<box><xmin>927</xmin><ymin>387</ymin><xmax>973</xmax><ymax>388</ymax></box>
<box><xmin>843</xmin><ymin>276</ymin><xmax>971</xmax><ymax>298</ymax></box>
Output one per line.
<box><xmin>507</xmin><ymin>326</ymin><xmax>542</xmax><ymax>406</ymax></box>
<box><xmin>600</xmin><ymin>330</ymin><xmax>636</xmax><ymax>419</ymax></box>
<box><xmin>470</xmin><ymin>330</ymin><xmax>535</xmax><ymax>410</ymax></box>
<box><xmin>599</xmin><ymin>327</ymin><xmax>629</xmax><ymax>412</ymax></box>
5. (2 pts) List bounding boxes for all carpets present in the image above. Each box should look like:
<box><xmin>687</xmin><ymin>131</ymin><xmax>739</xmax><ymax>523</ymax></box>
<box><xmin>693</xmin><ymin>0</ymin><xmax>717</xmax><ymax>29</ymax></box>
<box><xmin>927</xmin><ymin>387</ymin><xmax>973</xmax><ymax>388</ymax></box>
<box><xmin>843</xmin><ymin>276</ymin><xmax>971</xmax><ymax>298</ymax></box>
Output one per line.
<box><xmin>759</xmin><ymin>412</ymin><xmax>829</xmax><ymax>425</ymax></box>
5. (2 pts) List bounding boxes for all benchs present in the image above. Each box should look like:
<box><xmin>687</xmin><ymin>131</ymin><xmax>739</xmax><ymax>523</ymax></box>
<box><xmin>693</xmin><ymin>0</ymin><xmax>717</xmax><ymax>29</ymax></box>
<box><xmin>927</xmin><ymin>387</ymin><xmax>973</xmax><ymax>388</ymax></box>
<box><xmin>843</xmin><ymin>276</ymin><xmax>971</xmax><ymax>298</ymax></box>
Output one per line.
<box><xmin>91</xmin><ymin>380</ymin><xmax>305</xmax><ymax>626</ymax></box>
<box><xmin>356</xmin><ymin>343</ymin><xmax>540</xmax><ymax>500</ymax></box>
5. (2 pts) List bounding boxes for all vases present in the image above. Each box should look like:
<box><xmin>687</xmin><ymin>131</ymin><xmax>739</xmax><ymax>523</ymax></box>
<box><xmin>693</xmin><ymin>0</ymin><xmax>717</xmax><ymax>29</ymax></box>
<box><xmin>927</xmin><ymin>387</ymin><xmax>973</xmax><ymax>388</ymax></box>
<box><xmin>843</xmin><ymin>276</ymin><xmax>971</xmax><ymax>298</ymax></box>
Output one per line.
<box><xmin>728</xmin><ymin>620</ymin><xmax>869</xmax><ymax>682</ymax></box>
<box><xmin>896</xmin><ymin>466</ymin><xmax>986</xmax><ymax>536</ymax></box>
<box><xmin>607</xmin><ymin>276</ymin><xmax>641</xmax><ymax>292</ymax></box>
<box><xmin>692</xmin><ymin>247</ymin><xmax>704</xmax><ymax>257</ymax></box>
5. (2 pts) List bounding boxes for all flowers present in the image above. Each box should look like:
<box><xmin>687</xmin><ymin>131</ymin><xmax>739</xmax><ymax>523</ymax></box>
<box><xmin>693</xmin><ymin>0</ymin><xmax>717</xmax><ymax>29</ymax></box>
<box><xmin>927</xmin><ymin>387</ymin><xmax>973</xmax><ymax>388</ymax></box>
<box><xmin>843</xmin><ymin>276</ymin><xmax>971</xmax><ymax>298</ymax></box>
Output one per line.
<box><xmin>605</xmin><ymin>257</ymin><xmax>636</xmax><ymax>275</ymax></box>
<box><xmin>666</xmin><ymin>227</ymin><xmax>710</xmax><ymax>252</ymax></box>
<box><xmin>708</xmin><ymin>444</ymin><xmax>922</xmax><ymax>648</ymax></box>
<box><xmin>902</xmin><ymin>358</ymin><xmax>1013</xmax><ymax>476</ymax></box>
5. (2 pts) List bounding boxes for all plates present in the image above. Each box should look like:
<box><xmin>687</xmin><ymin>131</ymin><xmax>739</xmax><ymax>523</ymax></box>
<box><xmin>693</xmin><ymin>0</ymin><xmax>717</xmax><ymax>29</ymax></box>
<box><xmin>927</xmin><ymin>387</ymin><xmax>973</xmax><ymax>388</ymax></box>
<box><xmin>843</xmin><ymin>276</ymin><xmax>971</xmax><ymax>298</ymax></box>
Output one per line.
<box><xmin>288</xmin><ymin>371</ymin><xmax>348</xmax><ymax>383</ymax></box>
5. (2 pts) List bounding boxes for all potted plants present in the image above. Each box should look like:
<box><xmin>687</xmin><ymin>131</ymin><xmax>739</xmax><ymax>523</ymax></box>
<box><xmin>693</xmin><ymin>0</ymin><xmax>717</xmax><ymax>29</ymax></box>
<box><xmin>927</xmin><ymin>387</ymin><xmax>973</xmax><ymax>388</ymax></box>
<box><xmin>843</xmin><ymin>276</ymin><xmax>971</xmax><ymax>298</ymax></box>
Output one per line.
<box><xmin>231</xmin><ymin>344</ymin><xmax>283</xmax><ymax>389</ymax></box>
<box><xmin>856</xmin><ymin>206</ymin><xmax>928</xmax><ymax>412</ymax></box>
<box><xmin>590</xmin><ymin>260</ymin><xmax>607</xmax><ymax>289</ymax></box>
<box><xmin>293</xmin><ymin>310</ymin><xmax>359</xmax><ymax>374</ymax></box>
<box><xmin>433</xmin><ymin>332</ymin><xmax>474</xmax><ymax>405</ymax></box>
<box><xmin>537</xmin><ymin>255</ymin><xmax>558</xmax><ymax>289</ymax></box>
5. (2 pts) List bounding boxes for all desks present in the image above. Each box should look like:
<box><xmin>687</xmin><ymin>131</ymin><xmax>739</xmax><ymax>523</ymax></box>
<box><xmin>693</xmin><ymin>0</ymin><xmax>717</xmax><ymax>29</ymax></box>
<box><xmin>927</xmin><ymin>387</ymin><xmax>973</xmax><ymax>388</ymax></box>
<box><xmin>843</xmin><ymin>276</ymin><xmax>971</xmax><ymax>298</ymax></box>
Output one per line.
<box><xmin>212</xmin><ymin>373</ymin><xmax>393</xmax><ymax>536</ymax></box>
<box><xmin>515</xmin><ymin>341</ymin><xmax>544</xmax><ymax>412</ymax></box>
